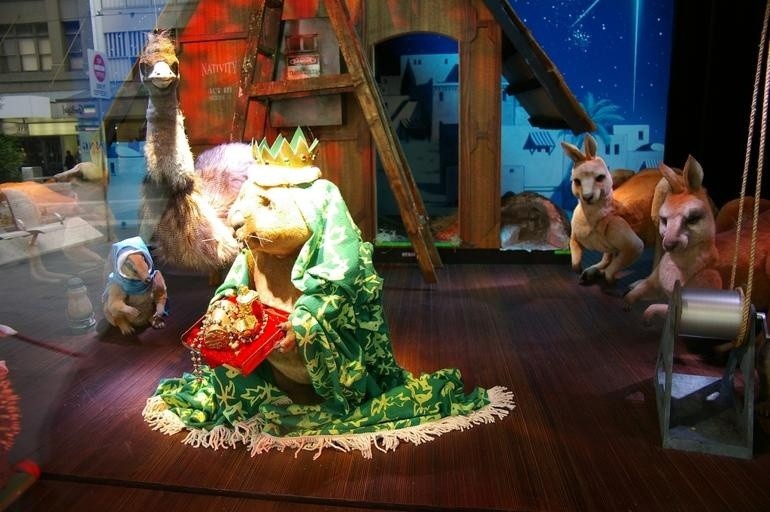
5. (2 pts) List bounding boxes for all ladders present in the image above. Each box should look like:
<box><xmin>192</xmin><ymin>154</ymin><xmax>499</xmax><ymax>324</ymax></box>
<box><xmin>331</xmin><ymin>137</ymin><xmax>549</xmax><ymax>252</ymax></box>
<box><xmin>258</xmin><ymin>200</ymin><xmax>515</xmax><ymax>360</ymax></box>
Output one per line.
<box><xmin>230</xmin><ymin>1</ymin><xmax>444</xmax><ymax>282</ymax></box>
